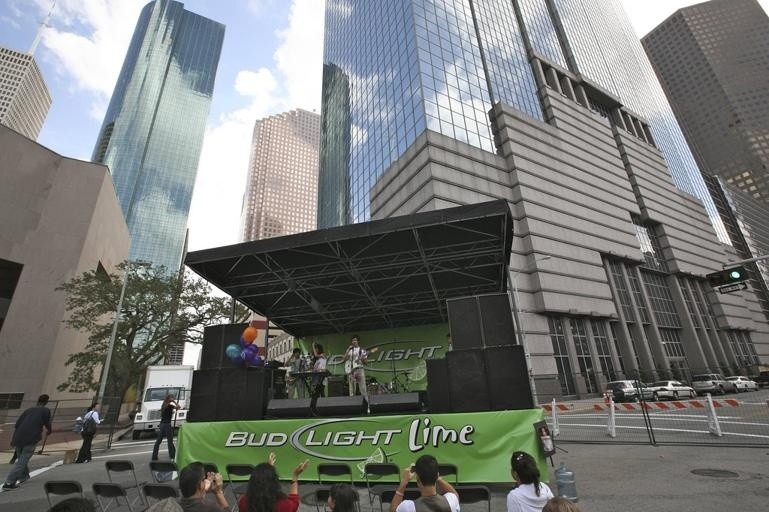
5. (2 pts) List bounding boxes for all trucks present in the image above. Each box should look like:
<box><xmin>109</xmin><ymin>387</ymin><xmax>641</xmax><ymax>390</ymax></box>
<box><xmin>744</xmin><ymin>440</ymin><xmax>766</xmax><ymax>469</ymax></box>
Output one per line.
<box><xmin>133</xmin><ymin>362</ymin><xmax>193</xmax><ymax>438</ymax></box>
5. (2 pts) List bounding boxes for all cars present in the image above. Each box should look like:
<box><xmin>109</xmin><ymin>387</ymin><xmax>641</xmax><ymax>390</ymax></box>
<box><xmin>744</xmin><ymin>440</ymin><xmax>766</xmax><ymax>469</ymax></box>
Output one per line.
<box><xmin>608</xmin><ymin>373</ymin><xmax>759</xmax><ymax>402</ymax></box>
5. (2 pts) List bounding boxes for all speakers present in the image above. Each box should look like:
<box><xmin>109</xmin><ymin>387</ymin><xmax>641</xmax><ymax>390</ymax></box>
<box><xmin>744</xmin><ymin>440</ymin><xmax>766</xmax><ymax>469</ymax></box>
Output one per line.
<box><xmin>426</xmin><ymin>359</ymin><xmax>448</xmax><ymax>413</ymax></box>
<box><xmin>478</xmin><ymin>293</ymin><xmax>517</xmax><ymax>349</ymax></box>
<box><xmin>267</xmin><ymin>399</ymin><xmax>316</xmax><ymax>417</ymax></box>
<box><xmin>243</xmin><ymin>368</ymin><xmax>273</xmax><ymax>420</ymax></box>
<box><xmin>446</xmin><ymin>296</ymin><xmax>485</xmax><ymax>351</ymax></box>
<box><xmin>483</xmin><ymin>345</ymin><xmax>534</xmax><ymax>411</ymax></box>
<box><xmin>316</xmin><ymin>395</ymin><xmax>368</xmax><ymax>416</ymax></box>
<box><xmin>200</xmin><ymin>324</ymin><xmax>223</xmax><ymax>370</ymax></box>
<box><xmin>219</xmin><ymin>324</ymin><xmax>250</xmax><ymax>368</ymax></box>
<box><xmin>369</xmin><ymin>392</ymin><xmax>423</xmax><ymax>414</ymax></box>
<box><xmin>214</xmin><ymin>368</ymin><xmax>248</xmax><ymax>421</ymax></box>
<box><xmin>187</xmin><ymin>370</ymin><xmax>218</xmax><ymax>422</ymax></box>
<box><xmin>446</xmin><ymin>349</ymin><xmax>491</xmax><ymax>412</ymax></box>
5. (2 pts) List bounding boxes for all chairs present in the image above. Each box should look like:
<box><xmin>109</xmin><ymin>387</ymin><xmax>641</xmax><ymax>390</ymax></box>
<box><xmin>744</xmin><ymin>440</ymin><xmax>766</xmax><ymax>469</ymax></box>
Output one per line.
<box><xmin>105</xmin><ymin>460</ymin><xmax>147</xmax><ymax>506</ymax></box>
<box><xmin>203</xmin><ymin>463</ymin><xmax>228</xmax><ymax>494</ymax></box>
<box><xmin>142</xmin><ymin>484</ymin><xmax>180</xmax><ymax>506</ymax></box>
<box><xmin>455</xmin><ymin>486</ymin><xmax>491</xmax><ymax>512</ymax></box>
<box><xmin>44</xmin><ymin>481</ymin><xmax>83</xmax><ymax>507</ymax></box>
<box><xmin>315</xmin><ymin>488</ymin><xmax>361</xmax><ymax>512</ymax></box>
<box><xmin>317</xmin><ymin>463</ymin><xmax>356</xmax><ymax>507</ymax></box>
<box><xmin>379</xmin><ymin>489</ymin><xmax>421</xmax><ymax>512</ymax></box>
<box><xmin>437</xmin><ymin>464</ymin><xmax>457</xmax><ymax>486</ymax></box>
<box><xmin>226</xmin><ymin>464</ymin><xmax>256</xmax><ymax>512</ymax></box>
<box><xmin>93</xmin><ymin>483</ymin><xmax>135</xmax><ymax>512</ymax></box>
<box><xmin>364</xmin><ymin>463</ymin><xmax>401</xmax><ymax>512</ymax></box>
<box><xmin>149</xmin><ymin>461</ymin><xmax>180</xmax><ymax>501</ymax></box>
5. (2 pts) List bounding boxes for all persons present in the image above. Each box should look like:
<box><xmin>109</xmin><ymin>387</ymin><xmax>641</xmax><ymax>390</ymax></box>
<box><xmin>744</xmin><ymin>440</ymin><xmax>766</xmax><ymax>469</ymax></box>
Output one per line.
<box><xmin>391</xmin><ymin>453</ymin><xmax>462</xmax><ymax>512</ymax></box>
<box><xmin>344</xmin><ymin>334</ymin><xmax>372</xmax><ymax>415</ymax></box>
<box><xmin>238</xmin><ymin>451</ymin><xmax>310</xmax><ymax>512</ymax></box>
<box><xmin>327</xmin><ymin>481</ymin><xmax>361</xmax><ymax>511</ymax></box>
<box><xmin>445</xmin><ymin>333</ymin><xmax>452</xmax><ymax>352</ymax></box>
<box><xmin>506</xmin><ymin>451</ymin><xmax>555</xmax><ymax>511</ymax></box>
<box><xmin>151</xmin><ymin>393</ymin><xmax>182</xmax><ymax>463</ymax></box>
<box><xmin>287</xmin><ymin>348</ymin><xmax>307</xmax><ymax>398</ymax></box>
<box><xmin>174</xmin><ymin>461</ymin><xmax>232</xmax><ymax>512</ymax></box>
<box><xmin>74</xmin><ymin>402</ymin><xmax>105</xmax><ymax>464</ymax></box>
<box><xmin>308</xmin><ymin>342</ymin><xmax>332</xmax><ymax>398</ymax></box>
<box><xmin>1</xmin><ymin>394</ymin><xmax>52</xmax><ymax>491</ymax></box>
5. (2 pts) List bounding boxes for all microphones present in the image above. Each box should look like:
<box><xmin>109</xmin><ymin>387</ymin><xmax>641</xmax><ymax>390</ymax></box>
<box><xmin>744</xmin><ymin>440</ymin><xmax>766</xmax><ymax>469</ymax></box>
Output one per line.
<box><xmin>352</xmin><ymin>343</ymin><xmax>354</xmax><ymax>349</ymax></box>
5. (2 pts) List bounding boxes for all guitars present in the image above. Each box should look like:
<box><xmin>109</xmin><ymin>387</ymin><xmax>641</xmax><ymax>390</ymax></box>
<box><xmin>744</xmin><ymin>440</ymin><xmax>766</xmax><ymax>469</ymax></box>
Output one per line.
<box><xmin>345</xmin><ymin>347</ymin><xmax>378</xmax><ymax>375</ymax></box>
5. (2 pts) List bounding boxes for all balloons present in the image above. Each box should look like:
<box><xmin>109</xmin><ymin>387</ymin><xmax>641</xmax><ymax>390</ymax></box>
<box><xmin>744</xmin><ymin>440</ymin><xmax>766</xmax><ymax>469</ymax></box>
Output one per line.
<box><xmin>225</xmin><ymin>326</ymin><xmax>263</xmax><ymax>365</ymax></box>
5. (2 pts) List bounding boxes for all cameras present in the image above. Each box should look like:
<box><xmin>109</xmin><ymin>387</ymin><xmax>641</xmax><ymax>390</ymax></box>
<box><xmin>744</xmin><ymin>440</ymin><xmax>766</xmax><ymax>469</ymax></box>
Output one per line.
<box><xmin>412</xmin><ymin>466</ymin><xmax>417</xmax><ymax>472</ymax></box>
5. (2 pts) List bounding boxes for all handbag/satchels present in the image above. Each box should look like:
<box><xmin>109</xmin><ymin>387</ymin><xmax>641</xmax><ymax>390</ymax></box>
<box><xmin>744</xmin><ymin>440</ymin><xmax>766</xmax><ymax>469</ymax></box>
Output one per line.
<box><xmin>83</xmin><ymin>412</ymin><xmax>96</xmax><ymax>433</ymax></box>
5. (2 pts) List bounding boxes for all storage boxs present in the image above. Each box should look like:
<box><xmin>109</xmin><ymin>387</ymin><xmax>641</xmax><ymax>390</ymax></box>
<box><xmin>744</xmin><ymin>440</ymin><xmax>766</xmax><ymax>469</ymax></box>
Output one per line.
<box><xmin>63</xmin><ymin>449</ymin><xmax>77</xmax><ymax>464</ymax></box>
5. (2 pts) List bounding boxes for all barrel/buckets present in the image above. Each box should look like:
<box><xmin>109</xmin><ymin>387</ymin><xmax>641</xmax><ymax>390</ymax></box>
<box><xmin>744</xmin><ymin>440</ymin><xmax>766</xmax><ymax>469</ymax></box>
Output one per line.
<box><xmin>555</xmin><ymin>462</ymin><xmax>578</xmax><ymax>504</ymax></box>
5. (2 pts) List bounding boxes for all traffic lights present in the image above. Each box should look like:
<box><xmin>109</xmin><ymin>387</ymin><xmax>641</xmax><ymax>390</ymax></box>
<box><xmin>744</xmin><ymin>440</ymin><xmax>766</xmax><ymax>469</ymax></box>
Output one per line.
<box><xmin>706</xmin><ymin>264</ymin><xmax>747</xmax><ymax>289</ymax></box>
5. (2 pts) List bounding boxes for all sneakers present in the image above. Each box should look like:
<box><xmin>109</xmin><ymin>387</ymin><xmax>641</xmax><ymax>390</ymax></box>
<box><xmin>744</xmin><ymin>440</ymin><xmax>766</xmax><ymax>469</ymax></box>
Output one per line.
<box><xmin>19</xmin><ymin>475</ymin><xmax>30</xmax><ymax>484</ymax></box>
<box><xmin>2</xmin><ymin>481</ymin><xmax>20</xmax><ymax>491</ymax></box>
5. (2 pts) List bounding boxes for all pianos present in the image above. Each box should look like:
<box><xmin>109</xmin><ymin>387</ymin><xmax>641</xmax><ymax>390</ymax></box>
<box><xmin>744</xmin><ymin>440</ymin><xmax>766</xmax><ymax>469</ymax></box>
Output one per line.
<box><xmin>290</xmin><ymin>370</ymin><xmax>331</xmax><ymax>377</ymax></box>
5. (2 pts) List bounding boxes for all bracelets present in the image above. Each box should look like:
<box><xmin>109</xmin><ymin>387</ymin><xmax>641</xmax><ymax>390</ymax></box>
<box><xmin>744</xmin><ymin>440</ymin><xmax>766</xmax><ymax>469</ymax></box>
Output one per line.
<box><xmin>290</xmin><ymin>480</ymin><xmax>298</xmax><ymax>485</ymax></box>
<box><xmin>436</xmin><ymin>477</ymin><xmax>443</xmax><ymax>482</ymax></box>
<box><xmin>395</xmin><ymin>490</ymin><xmax>404</xmax><ymax>497</ymax></box>
